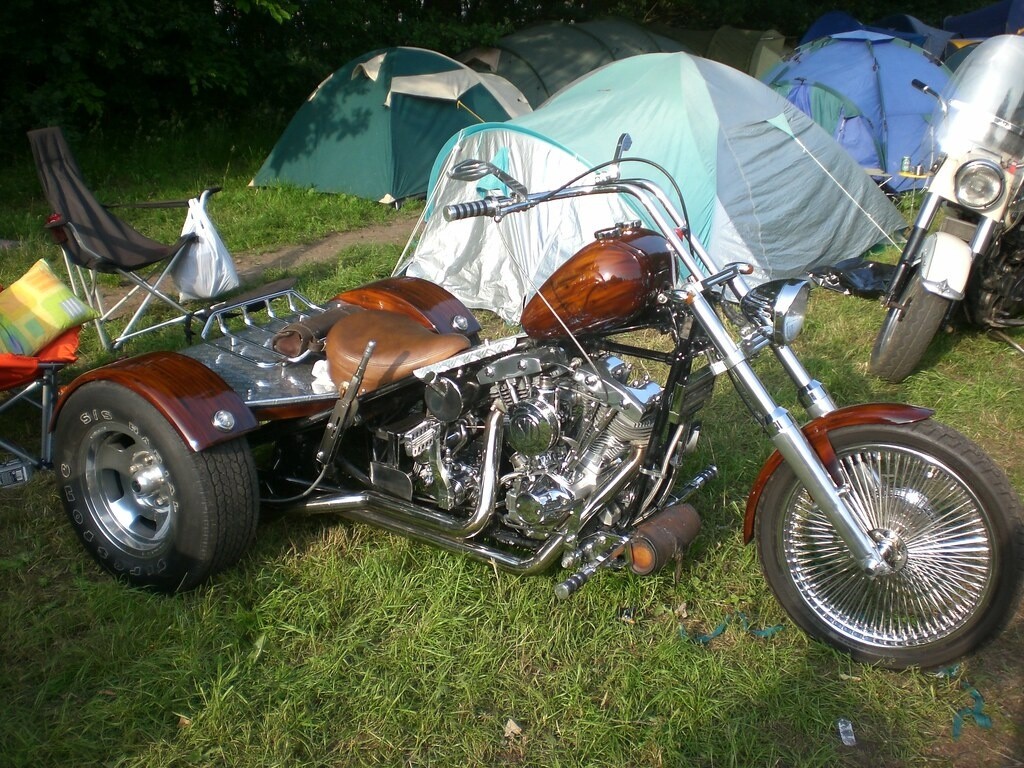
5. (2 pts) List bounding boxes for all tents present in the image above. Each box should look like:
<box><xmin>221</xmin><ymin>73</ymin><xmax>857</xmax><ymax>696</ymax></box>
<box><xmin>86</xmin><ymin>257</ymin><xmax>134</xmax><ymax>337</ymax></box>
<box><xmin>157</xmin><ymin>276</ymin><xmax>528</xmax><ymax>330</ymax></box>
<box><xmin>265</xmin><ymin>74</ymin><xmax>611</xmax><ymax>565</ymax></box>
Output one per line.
<box><xmin>393</xmin><ymin>50</ymin><xmax>912</xmax><ymax>325</ymax></box>
<box><xmin>247</xmin><ymin>0</ymin><xmax>1024</xmax><ymax>214</ymax></box>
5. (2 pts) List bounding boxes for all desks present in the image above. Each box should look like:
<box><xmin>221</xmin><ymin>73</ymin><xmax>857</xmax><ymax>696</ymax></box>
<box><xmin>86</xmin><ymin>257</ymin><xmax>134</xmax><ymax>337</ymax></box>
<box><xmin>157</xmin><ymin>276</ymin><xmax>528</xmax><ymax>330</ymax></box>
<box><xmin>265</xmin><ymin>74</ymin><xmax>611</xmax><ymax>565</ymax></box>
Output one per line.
<box><xmin>900</xmin><ymin>172</ymin><xmax>927</xmax><ymax>219</ymax></box>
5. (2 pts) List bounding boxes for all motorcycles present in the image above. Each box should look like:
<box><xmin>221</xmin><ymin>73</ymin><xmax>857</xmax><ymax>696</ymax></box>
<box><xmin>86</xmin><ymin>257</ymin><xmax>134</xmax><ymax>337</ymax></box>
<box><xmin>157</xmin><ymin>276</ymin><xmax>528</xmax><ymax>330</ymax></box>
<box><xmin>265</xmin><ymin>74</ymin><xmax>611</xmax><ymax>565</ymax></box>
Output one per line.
<box><xmin>869</xmin><ymin>32</ymin><xmax>1024</xmax><ymax>384</ymax></box>
<box><xmin>42</xmin><ymin>132</ymin><xmax>1024</xmax><ymax>666</ymax></box>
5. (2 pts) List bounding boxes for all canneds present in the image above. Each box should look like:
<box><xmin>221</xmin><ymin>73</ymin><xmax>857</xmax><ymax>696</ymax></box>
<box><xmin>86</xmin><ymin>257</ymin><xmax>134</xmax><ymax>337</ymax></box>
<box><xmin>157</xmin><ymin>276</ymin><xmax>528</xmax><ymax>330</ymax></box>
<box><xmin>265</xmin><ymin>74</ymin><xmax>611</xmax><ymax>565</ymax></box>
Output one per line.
<box><xmin>901</xmin><ymin>156</ymin><xmax>911</xmax><ymax>172</ymax></box>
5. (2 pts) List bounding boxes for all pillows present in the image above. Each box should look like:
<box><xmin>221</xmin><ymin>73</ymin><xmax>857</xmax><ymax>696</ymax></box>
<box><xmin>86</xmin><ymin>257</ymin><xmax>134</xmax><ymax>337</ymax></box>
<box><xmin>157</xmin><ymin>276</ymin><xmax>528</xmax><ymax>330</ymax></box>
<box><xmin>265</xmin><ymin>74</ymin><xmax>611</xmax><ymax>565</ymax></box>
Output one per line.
<box><xmin>0</xmin><ymin>258</ymin><xmax>102</xmax><ymax>356</ymax></box>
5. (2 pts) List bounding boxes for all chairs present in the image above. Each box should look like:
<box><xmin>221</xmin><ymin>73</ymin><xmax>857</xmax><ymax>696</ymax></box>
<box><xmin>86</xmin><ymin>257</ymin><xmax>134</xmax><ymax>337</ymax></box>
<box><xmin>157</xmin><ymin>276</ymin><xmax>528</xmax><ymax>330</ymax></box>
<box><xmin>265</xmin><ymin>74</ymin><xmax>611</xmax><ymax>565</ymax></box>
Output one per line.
<box><xmin>27</xmin><ymin>126</ymin><xmax>225</xmax><ymax>351</ymax></box>
<box><xmin>864</xmin><ymin>169</ymin><xmax>892</xmax><ymax>188</ymax></box>
<box><xmin>0</xmin><ymin>280</ymin><xmax>81</xmax><ymax>471</ymax></box>
